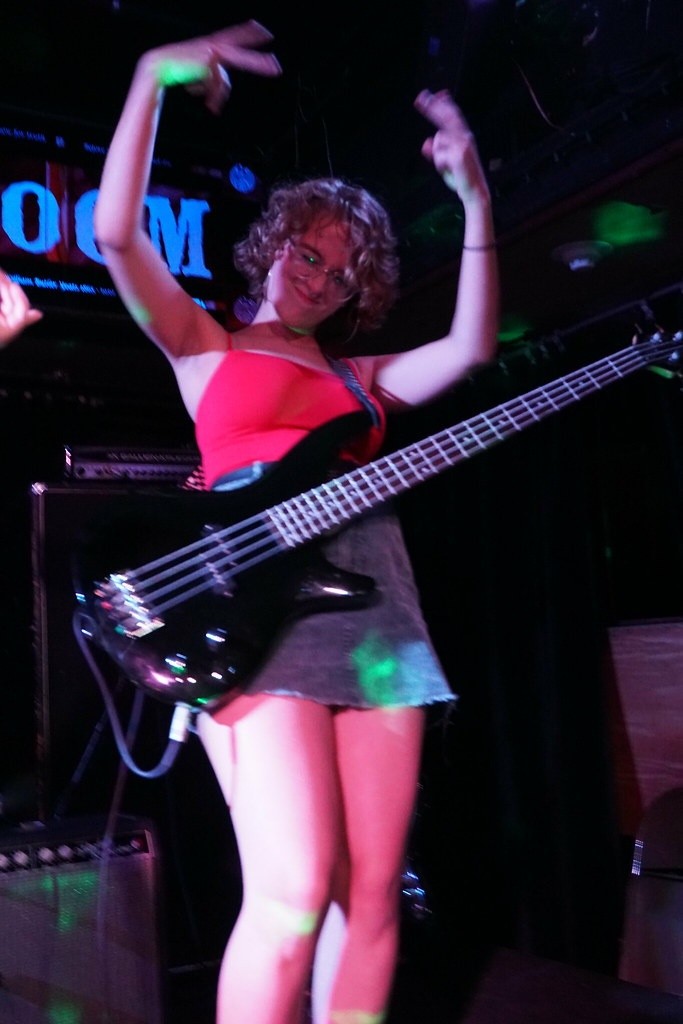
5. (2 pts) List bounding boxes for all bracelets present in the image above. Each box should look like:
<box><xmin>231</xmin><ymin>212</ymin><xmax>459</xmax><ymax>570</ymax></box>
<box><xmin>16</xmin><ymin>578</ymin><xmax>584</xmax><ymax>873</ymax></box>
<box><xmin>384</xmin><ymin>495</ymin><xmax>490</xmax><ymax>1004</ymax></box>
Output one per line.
<box><xmin>462</xmin><ymin>243</ymin><xmax>498</xmax><ymax>251</ymax></box>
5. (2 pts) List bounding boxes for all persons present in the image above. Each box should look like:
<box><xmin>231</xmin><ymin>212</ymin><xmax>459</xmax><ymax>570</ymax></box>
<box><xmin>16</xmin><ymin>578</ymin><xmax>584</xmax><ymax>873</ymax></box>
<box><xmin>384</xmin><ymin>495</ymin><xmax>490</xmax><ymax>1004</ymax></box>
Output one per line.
<box><xmin>94</xmin><ymin>21</ymin><xmax>498</xmax><ymax>1024</ymax></box>
<box><xmin>0</xmin><ymin>268</ymin><xmax>43</xmax><ymax>349</ymax></box>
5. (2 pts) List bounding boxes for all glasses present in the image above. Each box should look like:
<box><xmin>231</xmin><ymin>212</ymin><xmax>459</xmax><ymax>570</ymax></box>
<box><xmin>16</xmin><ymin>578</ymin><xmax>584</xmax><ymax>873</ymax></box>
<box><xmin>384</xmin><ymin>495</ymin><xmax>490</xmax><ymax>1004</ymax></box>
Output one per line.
<box><xmin>286</xmin><ymin>235</ymin><xmax>362</xmax><ymax>303</ymax></box>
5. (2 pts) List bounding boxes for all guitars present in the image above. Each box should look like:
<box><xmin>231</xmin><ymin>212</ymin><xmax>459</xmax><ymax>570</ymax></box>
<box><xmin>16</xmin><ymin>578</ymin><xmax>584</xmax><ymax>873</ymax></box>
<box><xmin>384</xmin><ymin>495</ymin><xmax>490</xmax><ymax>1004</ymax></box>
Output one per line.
<box><xmin>69</xmin><ymin>325</ymin><xmax>683</xmax><ymax>707</ymax></box>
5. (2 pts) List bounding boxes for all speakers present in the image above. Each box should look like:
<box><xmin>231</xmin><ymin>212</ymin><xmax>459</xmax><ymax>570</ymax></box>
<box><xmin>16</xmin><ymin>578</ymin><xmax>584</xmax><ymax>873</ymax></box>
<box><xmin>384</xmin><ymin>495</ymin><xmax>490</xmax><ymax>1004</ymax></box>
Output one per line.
<box><xmin>31</xmin><ymin>484</ymin><xmax>246</xmax><ymax>979</ymax></box>
<box><xmin>0</xmin><ymin>813</ymin><xmax>167</xmax><ymax>1024</ymax></box>
